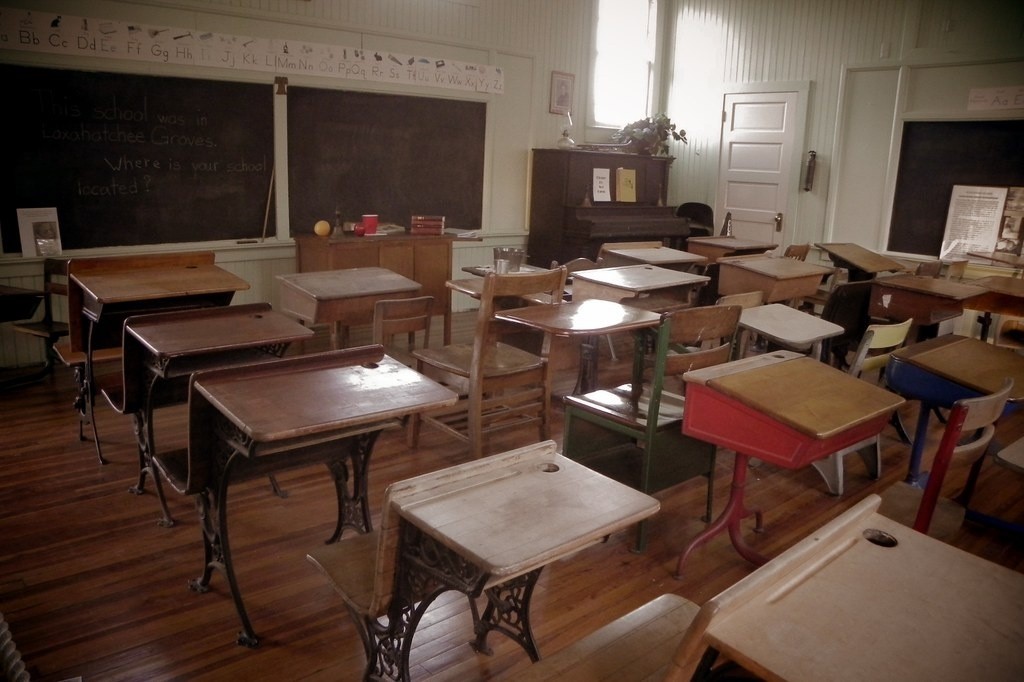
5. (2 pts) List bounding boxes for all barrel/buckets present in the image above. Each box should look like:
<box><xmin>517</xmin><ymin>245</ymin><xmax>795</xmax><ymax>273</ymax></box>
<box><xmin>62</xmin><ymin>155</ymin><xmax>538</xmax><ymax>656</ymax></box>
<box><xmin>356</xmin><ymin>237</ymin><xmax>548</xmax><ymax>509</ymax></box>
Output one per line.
<box><xmin>494</xmin><ymin>246</ymin><xmax>526</xmax><ymax>272</ymax></box>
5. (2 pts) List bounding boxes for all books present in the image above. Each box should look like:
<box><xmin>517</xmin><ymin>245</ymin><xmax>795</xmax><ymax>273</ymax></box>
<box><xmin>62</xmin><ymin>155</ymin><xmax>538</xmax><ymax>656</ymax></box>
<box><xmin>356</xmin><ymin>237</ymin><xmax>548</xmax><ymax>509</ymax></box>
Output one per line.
<box><xmin>616</xmin><ymin>168</ymin><xmax>636</xmax><ymax>202</ymax></box>
<box><xmin>16</xmin><ymin>207</ymin><xmax>62</xmax><ymax>258</ymax></box>
<box><xmin>376</xmin><ymin>215</ymin><xmax>480</xmax><ymax>239</ymax></box>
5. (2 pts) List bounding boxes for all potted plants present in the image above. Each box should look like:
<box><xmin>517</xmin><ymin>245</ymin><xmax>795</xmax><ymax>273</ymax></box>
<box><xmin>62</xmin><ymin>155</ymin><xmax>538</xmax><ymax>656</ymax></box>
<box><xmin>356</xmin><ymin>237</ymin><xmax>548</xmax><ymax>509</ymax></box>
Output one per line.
<box><xmin>611</xmin><ymin>107</ymin><xmax>689</xmax><ymax>161</ymax></box>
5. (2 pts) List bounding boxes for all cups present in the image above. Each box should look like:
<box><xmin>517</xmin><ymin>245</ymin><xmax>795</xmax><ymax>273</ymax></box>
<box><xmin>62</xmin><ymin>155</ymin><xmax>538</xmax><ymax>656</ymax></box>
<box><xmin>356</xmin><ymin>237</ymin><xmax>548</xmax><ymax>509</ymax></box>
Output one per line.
<box><xmin>361</xmin><ymin>214</ymin><xmax>379</xmax><ymax>234</ymax></box>
<box><xmin>493</xmin><ymin>259</ymin><xmax>511</xmax><ymax>275</ymax></box>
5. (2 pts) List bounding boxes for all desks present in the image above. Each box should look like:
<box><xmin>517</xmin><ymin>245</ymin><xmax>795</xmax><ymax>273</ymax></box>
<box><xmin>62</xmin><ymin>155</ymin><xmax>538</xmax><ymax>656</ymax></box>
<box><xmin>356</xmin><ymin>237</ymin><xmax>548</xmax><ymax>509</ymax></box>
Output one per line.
<box><xmin>54</xmin><ymin>227</ymin><xmax>1024</xmax><ymax>682</ymax></box>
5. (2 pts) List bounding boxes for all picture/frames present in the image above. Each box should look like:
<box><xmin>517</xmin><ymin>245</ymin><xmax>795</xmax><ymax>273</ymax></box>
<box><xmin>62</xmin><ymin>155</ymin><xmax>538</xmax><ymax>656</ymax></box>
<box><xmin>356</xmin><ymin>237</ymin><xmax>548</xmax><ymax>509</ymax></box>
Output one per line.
<box><xmin>547</xmin><ymin>69</ymin><xmax>577</xmax><ymax>117</ymax></box>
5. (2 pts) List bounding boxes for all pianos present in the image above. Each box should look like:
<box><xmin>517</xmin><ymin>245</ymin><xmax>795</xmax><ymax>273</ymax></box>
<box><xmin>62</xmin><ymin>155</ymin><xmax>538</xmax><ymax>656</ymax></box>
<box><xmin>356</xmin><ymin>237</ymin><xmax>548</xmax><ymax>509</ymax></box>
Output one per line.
<box><xmin>526</xmin><ymin>147</ymin><xmax>692</xmax><ymax>268</ymax></box>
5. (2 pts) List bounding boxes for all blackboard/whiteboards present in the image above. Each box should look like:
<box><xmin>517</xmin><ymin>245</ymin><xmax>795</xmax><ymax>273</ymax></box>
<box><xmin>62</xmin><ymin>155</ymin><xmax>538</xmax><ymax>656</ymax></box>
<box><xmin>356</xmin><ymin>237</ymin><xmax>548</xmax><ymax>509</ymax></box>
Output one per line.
<box><xmin>1</xmin><ymin>62</ymin><xmax>277</xmax><ymax>258</ymax></box>
<box><xmin>284</xmin><ymin>79</ymin><xmax>487</xmax><ymax>243</ymax></box>
<box><xmin>881</xmin><ymin>113</ymin><xmax>1024</xmax><ymax>257</ymax></box>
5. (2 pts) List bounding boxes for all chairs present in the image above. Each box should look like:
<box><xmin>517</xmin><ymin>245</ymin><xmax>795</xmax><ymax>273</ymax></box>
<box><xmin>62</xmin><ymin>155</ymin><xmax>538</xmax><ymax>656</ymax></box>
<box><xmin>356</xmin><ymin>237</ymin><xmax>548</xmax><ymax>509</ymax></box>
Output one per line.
<box><xmin>12</xmin><ymin>258</ymin><xmax>74</xmax><ymax>372</ymax></box>
